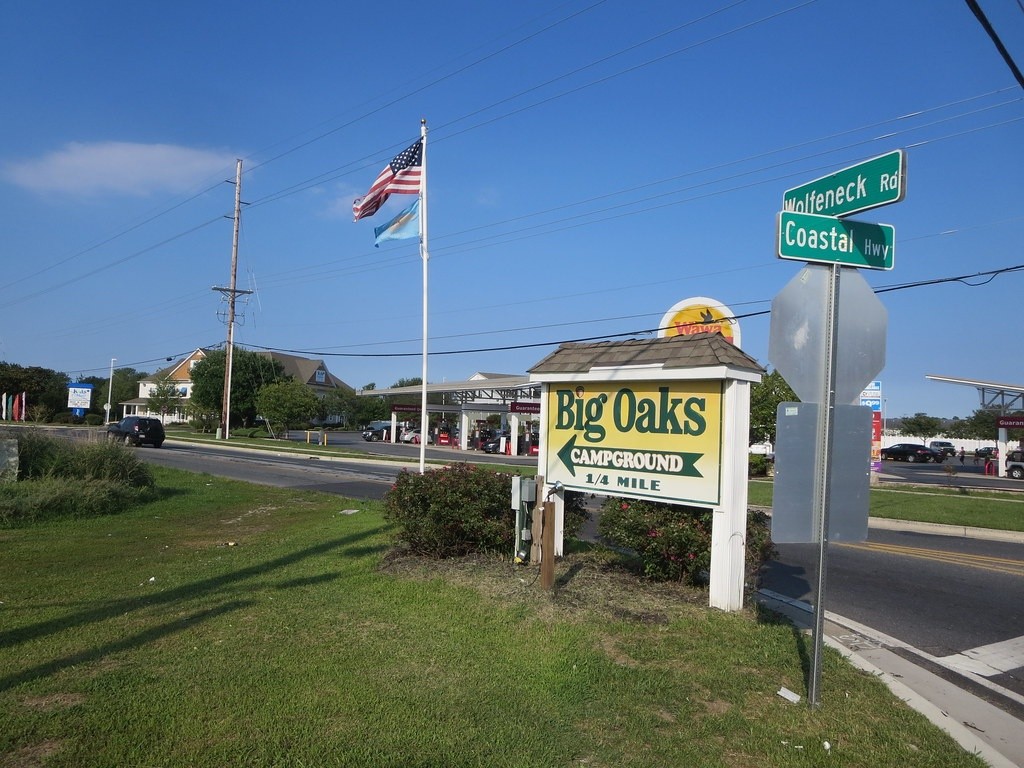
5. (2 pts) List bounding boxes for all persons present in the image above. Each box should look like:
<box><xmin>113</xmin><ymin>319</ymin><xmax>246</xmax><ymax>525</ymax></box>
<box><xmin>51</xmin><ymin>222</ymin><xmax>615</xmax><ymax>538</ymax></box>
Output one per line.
<box><xmin>974</xmin><ymin>448</ymin><xmax>979</xmax><ymax>464</ymax></box>
<box><xmin>959</xmin><ymin>447</ymin><xmax>965</xmax><ymax>466</ymax></box>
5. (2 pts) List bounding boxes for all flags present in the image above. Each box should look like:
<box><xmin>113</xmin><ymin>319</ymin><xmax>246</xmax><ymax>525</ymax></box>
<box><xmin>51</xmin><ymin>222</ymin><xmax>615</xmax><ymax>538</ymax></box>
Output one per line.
<box><xmin>352</xmin><ymin>136</ymin><xmax>423</xmax><ymax>223</ymax></box>
<box><xmin>374</xmin><ymin>198</ymin><xmax>419</xmax><ymax>248</ymax></box>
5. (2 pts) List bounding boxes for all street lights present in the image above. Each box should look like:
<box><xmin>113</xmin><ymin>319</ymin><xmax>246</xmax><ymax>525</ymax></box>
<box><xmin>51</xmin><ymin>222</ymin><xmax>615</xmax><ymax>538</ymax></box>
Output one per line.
<box><xmin>883</xmin><ymin>398</ymin><xmax>887</xmax><ymax>448</ymax></box>
<box><xmin>107</xmin><ymin>359</ymin><xmax>118</xmax><ymax>423</ymax></box>
<box><xmin>442</xmin><ymin>376</ymin><xmax>447</xmax><ymax>422</ymax></box>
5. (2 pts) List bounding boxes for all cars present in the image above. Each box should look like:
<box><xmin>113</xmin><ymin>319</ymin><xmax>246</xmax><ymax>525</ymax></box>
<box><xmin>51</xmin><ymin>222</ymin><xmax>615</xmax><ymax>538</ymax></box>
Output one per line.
<box><xmin>362</xmin><ymin>426</ymin><xmax>401</xmax><ymax>442</ymax></box>
<box><xmin>401</xmin><ymin>429</ymin><xmax>434</xmax><ymax>444</ymax></box>
<box><xmin>930</xmin><ymin>441</ymin><xmax>958</xmax><ymax>457</ymax></box>
<box><xmin>481</xmin><ymin>435</ymin><xmax>523</xmax><ymax>454</ymax></box>
<box><xmin>880</xmin><ymin>444</ymin><xmax>930</xmax><ymax>463</ymax></box>
<box><xmin>1004</xmin><ymin>450</ymin><xmax>1024</xmax><ymax>479</ymax></box>
<box><xmin>977</xmin><ymin>447</ymin><xmax>999</xmax><ymax>458</ymax></box>
<box><xmin>106</xmin><ymin>416</ymin><xmax>165</xmax><ymax>447</ymax></box>
